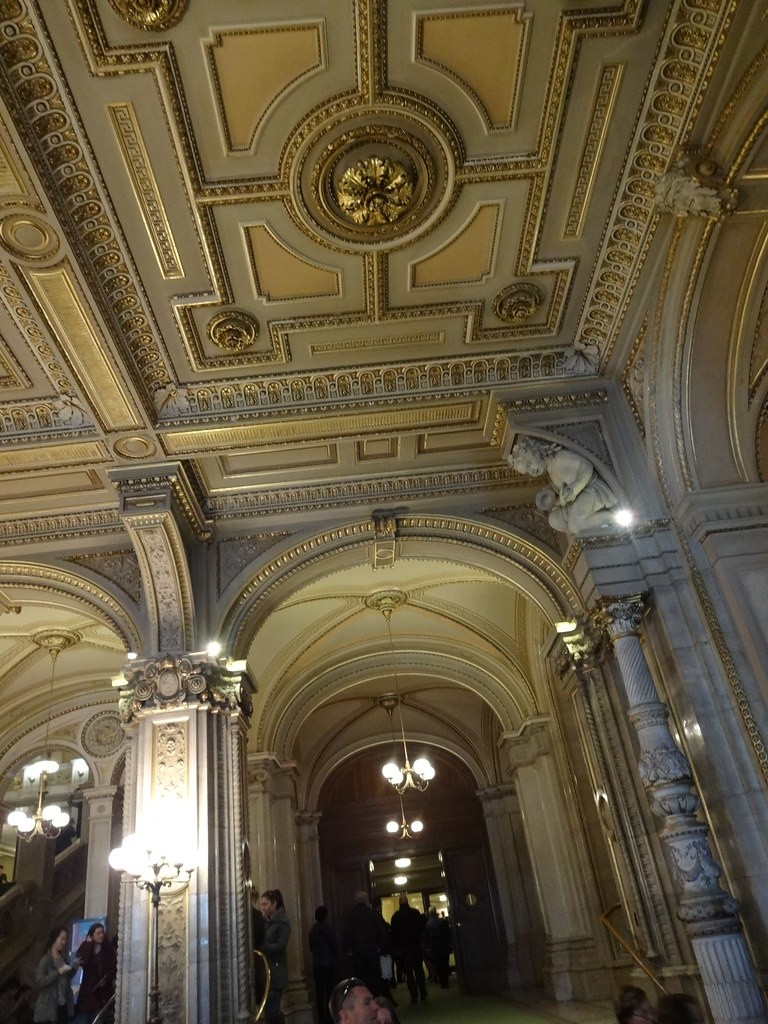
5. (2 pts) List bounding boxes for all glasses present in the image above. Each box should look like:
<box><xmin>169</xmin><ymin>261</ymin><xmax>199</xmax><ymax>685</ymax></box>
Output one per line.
<box><xmin>338</xmin><ymin>976</ymin><xmax>359</xmax><ymax>1010</ymax></box>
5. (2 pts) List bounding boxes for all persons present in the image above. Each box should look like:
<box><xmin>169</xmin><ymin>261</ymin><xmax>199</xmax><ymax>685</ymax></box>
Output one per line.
<box><xmin>309</xmin><ymin>889</ymin><xmax>451</xmax><ymax>1024</ymax></box>
<box><xmin>252</xmin><ymin>885</ymin><xmax>291</xmax><ymax>1024</ymax></box>
<box><xmin>614</xmin><ymin>984</ymin><xmax>705</xmax><ymax>1024</ymax></box>
<box><xmin>32</xmin><ymin>922</ymin><xmax>118</xmax><ymax>1024</ymax></box>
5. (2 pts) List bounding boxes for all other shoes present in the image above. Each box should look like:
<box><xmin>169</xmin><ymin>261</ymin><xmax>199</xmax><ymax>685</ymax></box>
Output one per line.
<box><xmin>420</xmin><ymin>990</ymin><xmax>429</xmax><ymax>1000</ymax></box>
<box><xmin>411</xmin><ymin>992</ymin><xmax>419</xmax><ymax>1004</ymax></box>
<box><xmin>391</xmin><ymin>1000</ymin><xmax>399</xmax><ymax>1007</ymax></box>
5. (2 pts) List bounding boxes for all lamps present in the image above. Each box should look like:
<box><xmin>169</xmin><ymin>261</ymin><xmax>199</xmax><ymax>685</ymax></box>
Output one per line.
<box><xmin>395</xmin><ymin>877</ymin><xmax>407</xmax><ymax>885</ymax></box>
<box><xmin>6</xmin><ymin>627</ymin><xmax>84</xmax><ymax>844</ymax></box>
<box><xmin>364</xmin><ymin>590</ymin><xmax>436</xmax><ymax>794</ymax></box>
<box><xmin>373</xmin><ymin>692</ymin><xmax>425</xmax><ymax>841</ymax></box>
<box><xmin>394</xmin><ymin>857</ymin><xmax>411</xmax><ymax>868</ymax></box>
<box><xmin>109</xmin><ymin>829</ymin><xmax>202</xmax><ymax>1024</ymax></box>
